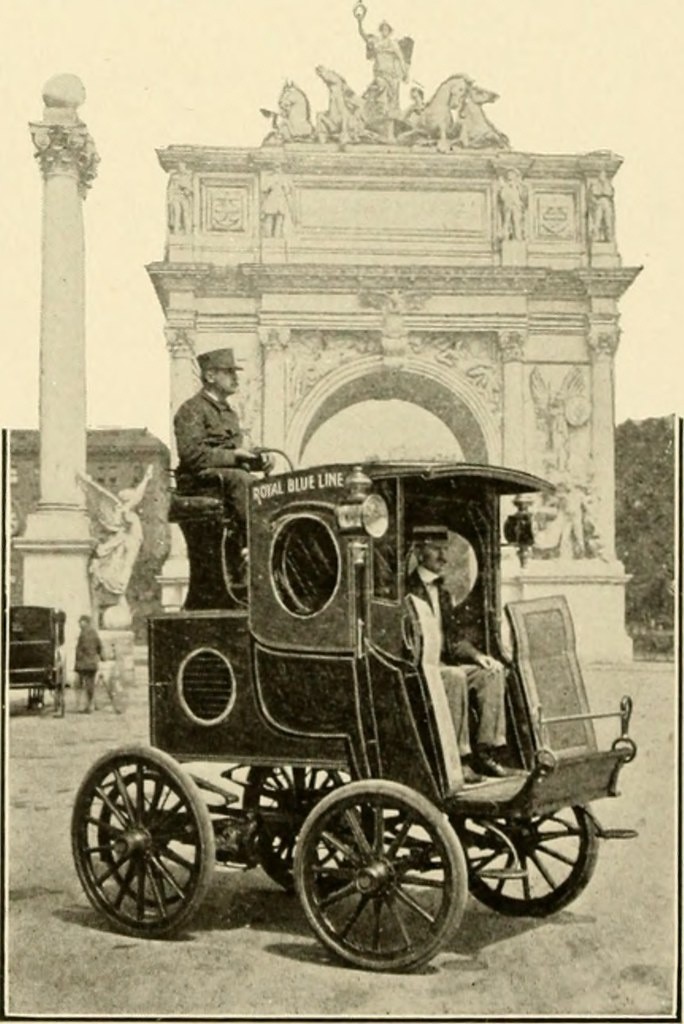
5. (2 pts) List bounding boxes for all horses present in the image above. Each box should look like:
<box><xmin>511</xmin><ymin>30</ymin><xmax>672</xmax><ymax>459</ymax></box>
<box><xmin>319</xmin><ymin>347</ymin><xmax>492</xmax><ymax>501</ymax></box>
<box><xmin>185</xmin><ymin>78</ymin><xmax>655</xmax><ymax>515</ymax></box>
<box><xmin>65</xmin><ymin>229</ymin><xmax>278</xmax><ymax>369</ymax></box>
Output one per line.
<box><xmin>258</xmin><ymin>63</ymin><xmax>364</xmax><ymax>146</ymax></box>
<box><xmin>414</xmin><ymin>76</ymin><xmax>510</xmax><ymax>151</ymax></box>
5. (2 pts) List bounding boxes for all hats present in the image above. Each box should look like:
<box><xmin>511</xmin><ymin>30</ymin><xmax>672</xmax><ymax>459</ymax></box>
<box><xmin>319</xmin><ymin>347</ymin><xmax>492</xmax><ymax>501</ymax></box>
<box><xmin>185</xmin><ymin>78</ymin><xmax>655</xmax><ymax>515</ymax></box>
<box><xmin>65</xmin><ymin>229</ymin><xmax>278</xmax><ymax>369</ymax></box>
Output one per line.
<box><xmin>197</xmin><ymin>349</ymin><xmax>244</xmax><ymax>371</ymax></box>
<box><xmin>406</xmin><ymin>526</ymin><xmax>456</xmax><ymax>547</ymax></box>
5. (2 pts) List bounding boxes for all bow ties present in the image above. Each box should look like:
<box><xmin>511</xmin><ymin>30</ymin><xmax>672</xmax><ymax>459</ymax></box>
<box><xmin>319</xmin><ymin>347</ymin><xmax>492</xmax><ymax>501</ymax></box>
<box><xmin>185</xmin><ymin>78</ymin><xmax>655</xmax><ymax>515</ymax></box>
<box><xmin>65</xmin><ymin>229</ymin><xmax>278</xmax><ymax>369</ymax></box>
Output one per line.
<box><xmin>432</xmin><ymin>578</ymin><xmax>444</xmax><ymax>585</ymax></box>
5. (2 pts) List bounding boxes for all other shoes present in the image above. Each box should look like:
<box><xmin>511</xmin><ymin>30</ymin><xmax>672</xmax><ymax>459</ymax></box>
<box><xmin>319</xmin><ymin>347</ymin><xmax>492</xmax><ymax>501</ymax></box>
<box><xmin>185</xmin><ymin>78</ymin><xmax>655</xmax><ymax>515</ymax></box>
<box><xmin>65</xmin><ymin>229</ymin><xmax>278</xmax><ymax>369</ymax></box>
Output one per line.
<box><xmin>473</xmin><ymin>752</ymin><xmax>507</xmax><ymax>777</ymax></box>
<box><xmin>461</xmin><ymin>763</ymin><xmax>481</xmax><ymax>783</ymax></box>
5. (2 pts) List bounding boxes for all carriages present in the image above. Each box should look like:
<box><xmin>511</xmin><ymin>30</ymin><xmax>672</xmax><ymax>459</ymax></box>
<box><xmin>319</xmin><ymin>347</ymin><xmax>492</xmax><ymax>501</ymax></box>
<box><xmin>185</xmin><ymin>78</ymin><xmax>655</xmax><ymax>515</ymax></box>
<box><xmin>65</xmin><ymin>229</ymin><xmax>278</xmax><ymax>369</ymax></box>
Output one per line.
<box><xmin>8</xmin><ymin>605</ymin><xmax>71</xmax><ymax>718</ymax></box>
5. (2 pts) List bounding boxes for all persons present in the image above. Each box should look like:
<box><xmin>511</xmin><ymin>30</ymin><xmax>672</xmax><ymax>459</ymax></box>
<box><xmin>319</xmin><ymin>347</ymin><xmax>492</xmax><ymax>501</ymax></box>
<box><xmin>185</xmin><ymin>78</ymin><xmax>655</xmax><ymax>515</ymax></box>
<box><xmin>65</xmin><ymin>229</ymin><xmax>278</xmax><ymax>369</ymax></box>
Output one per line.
<box><xmin>167</xmin><ymin>162</ymin><xmax>195</xmax><ymax>236</ymax></box>
<box><xmin>588</xmin><ymin>168</ymin><xmax>613</xmax><ymax>242</ymax></box>
<box><xmin>365</xmin><ymin>21</ymin><xmax>407</xmax><ymax>110</ymax></box>
<box><xmin>175</xmin><ymin>347</ymin><xmax>276</xmax><ymax>582</ymax></box>
<box><xmin>406</xmin><ymin>524</ymin><xmax>516</xmax><ymax>783</ymax></box>
<box><xmin>498</xmin><ymin>169</ymin><xmax>522</xmax><ymax>240</ymax></box>
<box><xmin>397</xmin><ymin>87</ymin><xmax>431</xmax><ymax>121</ymax></box>
<box><xmin>261</xmin><ymin>167</ymin><xmax>291</xmax><ymax>237</ymax></box>
<box><xmin>89</xmin><ymin>465</ymin><xmax>153</xmax><ymax>606</ymax></box>
<box><xmin>68</xmin><ymin>615</ymin><xmax>106</xmax><ymax>714</ymax></box>
